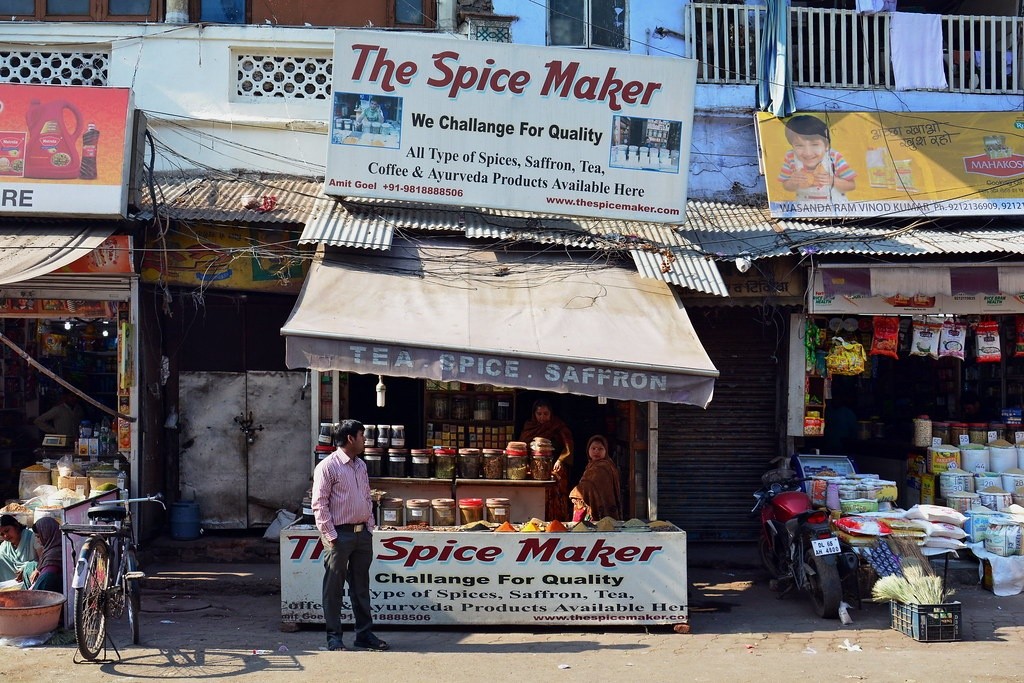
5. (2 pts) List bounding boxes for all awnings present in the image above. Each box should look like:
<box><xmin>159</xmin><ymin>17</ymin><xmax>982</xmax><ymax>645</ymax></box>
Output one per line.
<box><xmin>0</xmin><ymin>228</ymin><xmax>117</xmax><ymax>303</ymax></box>
<box><xmin>279</xmin><ymin>238</ymin><xmax>721</xmax><ymax>522</ymax></box>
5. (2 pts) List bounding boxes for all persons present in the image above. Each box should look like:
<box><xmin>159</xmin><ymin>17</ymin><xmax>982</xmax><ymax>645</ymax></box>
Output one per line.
<box><xmin>0</xmin><ymin>514</ymin><xmax>64</xmax><ymax>597</ymax></box>
<box><xmin>576</xmin><ymin>436</ymin><xmax>622</xmax><ymax>522</ymax></box>
<box><xmin>309</xmin><ymin>419</ymin><xmax>390</xmax><ymax>651</ymax></box>
<box><xmin>520</xmin><ymin>403</ymin><xmax>571</xmax><ymax>523</ymax></box>
<box><xmin>35</xmin><ymin>387</ymin><xmax>80</xmax><ymax>446</ymax></box>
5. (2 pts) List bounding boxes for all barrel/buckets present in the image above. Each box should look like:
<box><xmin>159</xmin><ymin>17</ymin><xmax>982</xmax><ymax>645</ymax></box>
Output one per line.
<box><xmin>171</xmin><ymin>503</ymin><xmax>201</xmax><ymax>540</ymax></box>
<box><xmin>336</xmin><ymin>119</ymin><xmax>391</xmax><ymax>135</ymax></box>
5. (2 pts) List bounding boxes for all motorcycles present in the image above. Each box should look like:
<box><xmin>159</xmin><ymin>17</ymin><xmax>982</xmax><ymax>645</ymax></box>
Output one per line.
<box><xmin>750</xmin><ymin>467</ymin><xmax>860</xmax><ymax>619</ymax></box>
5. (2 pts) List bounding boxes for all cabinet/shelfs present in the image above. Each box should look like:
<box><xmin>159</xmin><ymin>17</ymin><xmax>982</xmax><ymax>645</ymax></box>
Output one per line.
<box><xmin>37</xmin><ymin>319</ymin><xmax>118</xmax><ymax>440</ymax></box>
<box><xmin>931</xmin><ymin>332</ymin><xmax>1024</xmax><ymax>418</ymax></box>
<box><xmin>424</xmin><ymin>379</ymin><xmax>516</xmax><ymax>448</ymax></box>
<box><xmin>805</xmin><ymin>375</ymin><xmax>826</xmax><ymax>437</ymax></box>
<box><xmin>0</xmin><ymin>317</ymin><xmax>29</xmax><ymax>428</ymax></box>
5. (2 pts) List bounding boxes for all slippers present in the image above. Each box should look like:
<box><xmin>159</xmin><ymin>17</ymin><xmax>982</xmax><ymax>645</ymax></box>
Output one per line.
<box><xmin>353</xmin><ymin>639</ymin><xmax>390</xmax><ymax>650</ymax></box>
<box><xmin>329</xmin><ymin>643</ymin><xmax>346</xmax><ymax>650</ymax></box>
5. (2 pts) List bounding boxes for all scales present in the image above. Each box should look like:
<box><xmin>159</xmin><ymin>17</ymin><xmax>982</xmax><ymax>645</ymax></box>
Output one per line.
<box><xmin>42</xmin><ymin>434</ymin><xmax>73</xmax><ymax>447</ymax></box>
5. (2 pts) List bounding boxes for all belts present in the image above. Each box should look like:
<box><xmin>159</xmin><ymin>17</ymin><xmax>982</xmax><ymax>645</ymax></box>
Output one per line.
<box><xmin>335</xmin><ymin>523</ymin><xmax>367</xmax><ymax>533</ymax></box>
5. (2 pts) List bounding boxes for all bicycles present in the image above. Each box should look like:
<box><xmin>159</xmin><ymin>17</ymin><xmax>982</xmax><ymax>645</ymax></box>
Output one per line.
<box><xmin>60</xmin><ymin>491</ymin><xmax>167</xmax><ymax>666</ymax></box>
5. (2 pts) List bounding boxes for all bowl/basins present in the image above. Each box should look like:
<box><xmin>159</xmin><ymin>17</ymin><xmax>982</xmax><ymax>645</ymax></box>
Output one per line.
<box><xmin>0</xmin><ymin>590</ymin><xmax>68</xmax><ymax>635</ymax></box>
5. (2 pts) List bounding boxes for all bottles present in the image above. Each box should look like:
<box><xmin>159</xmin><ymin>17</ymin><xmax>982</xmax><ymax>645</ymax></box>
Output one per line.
<box><xmin>411</xmin><ymin>449</ymin><xmax>433</xmax><ymax>478</ymax></box>
<box><xmin>322</xmin><ymin>374</ymin><xmax>347</xmax><ymax>422</ymax></box>
<box><xmin>496</xmin><ymin>394</ymin><xmax>513</xmax><ymax>420</ymax></box>
<box><xmin>315</xmin><ymin>423</ymin><xmax>407</xmax><ymax>478</ymax></box>
<box><xmin>474</xmin><ymin>395</ymin><xmax>492</xmax><ymax>421</ymax></box>
<box><xmin>458</xmin><ymin>449</ymin><xmax>480</xmax><ymax>479</ymax></box>
<box><xmin>452</xmin><ymin>395</ymin><xmax>471</xmax><ymax>420</ymax></box>
<box><xmin>302</xmin><ymin>498</ymin><xmax>316</xmax><ymax>525</ymax></box>
<box><xmin>482</xmin><ymin>449</ymin><xmax>504</xmax><ymax>479</ymax></box>
<box><xmin>912</xmin><ymin>358</ymin><xmax>1023</xmax><ymax>446</ymax></box>
<box><xmin>23</xmin><ymin>99</ymin><xmax>100</xmax><ymax>179</ymax></box>
<box><xmin>431</xmin><ymin>394</ymin><xmax>448</xmax><ymax>420</ymax></box>
<box><xmin>857</xmin><ymin>416</ymin><xmax>884</xmax><ymax>439</ymax></box>
<box><xmin>66</xmin><ymin>318</ymin><xmax>117</xmax><ymax>393</ymax></box>
<box><xmin>78</xmin><ymin>416</ymin><xmax>117</xmax><ymax>455</ymax></box>
<box><xmin>371</xmin><ymin>499</ymin><xmax>511</xmax><ymax>527</ymax></box>
<box><xmin>506</xmin><ymin>451</ymin><xmax>552</xmax><ymax>480</ymax></box>
<box><xmin>435</xmin><ymin>449</ymin><xmax>457</xmax><ymax>479</ymax></box>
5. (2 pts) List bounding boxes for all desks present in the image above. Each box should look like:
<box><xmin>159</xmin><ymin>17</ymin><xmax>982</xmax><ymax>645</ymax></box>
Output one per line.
<box><xmin>368</xmin><ymin>477</ymin><xmax>453</xmax><ymax>527</ymax></box>
<box><xmin>455</xmin><ymin>478</ymin><xmax>557</xmax><ymax>523</ymax></box>
<box><xmin>838</xmin><ymin>540</ymin><xmax>950</xmax><ymax>610</ymax></box>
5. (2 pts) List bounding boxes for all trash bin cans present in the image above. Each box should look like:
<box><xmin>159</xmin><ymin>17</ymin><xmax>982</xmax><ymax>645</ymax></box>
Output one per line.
<box><xmin>172</xmin><ymin>502</ymin><xmax>201</xmax><ymax>538</ymax></box>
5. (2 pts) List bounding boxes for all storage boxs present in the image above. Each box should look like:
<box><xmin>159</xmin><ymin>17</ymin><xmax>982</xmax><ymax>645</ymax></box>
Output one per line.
<box><xmin>889</xmin><ymin>599</ymin><xmax>962</xmax><ymax>641</ymax></box>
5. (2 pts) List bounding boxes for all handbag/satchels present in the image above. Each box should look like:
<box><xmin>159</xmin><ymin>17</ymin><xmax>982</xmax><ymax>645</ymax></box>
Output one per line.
<box><xmin>263</xmin><ymin>509</ymin><xmax>296</xmax><ymax>539</ymax></box>
